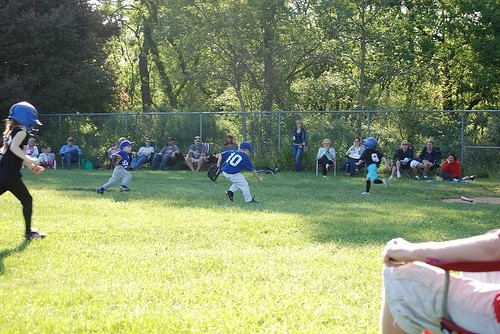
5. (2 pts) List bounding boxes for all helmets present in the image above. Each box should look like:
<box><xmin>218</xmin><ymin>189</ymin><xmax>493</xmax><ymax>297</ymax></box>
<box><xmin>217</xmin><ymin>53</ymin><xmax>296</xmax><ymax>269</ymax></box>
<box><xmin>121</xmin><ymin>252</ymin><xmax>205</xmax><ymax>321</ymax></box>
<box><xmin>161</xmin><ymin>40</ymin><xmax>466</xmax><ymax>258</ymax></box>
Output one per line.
<box><xmin>7</xmin><ymin>101</ymin><xmax>44</xmax><ymax>128</ymax></box>
<box><xmin>363</xmin><ymin>136</ymin><xmax>378</xmax><ymax>150</ymax></box>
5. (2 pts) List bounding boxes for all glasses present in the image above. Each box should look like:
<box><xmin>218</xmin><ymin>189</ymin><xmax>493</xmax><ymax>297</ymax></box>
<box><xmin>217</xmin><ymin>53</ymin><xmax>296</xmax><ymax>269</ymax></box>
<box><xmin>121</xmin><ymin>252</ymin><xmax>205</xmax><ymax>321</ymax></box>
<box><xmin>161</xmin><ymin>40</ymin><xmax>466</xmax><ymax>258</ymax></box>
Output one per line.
<box><xmin>403</xmin><ymin>144</ymin><xmax>408</xmax><ymax>145</ymax></box>
<box><xmin>69</xmin><ymin>141</ymin><xmax>73</xmax><ymax>142</ymax></box>
<box><xmin>324</xmin><ymin>143</ymin><xmax>330</xmax><ymax>144</ymax></box>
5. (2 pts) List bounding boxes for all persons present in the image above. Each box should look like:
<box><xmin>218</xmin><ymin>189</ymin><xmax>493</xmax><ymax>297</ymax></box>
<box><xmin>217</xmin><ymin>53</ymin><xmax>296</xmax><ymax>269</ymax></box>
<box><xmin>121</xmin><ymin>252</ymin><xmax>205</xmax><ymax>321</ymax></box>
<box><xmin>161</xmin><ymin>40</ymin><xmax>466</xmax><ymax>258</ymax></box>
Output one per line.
<box><xmin>22</xmin><ymin>138</ymin><xmax>38</xmax><ymax>157</ymax></box>
<box><xmin>292</xmin><ymin>120</ymin><xmax>308</xmax><ymax>172</ymax></box>
<box><xmin>0</xmin><ymin>101</ymin><xmax>48</xmax><ymax>241</ymax></box>
<box><xmin>39</xmin><ymin>146</ymin><xmax>55</xmax><ymax>169</ymax></box>
<box><xmin>441</xmin><ymin>153</ymin><xmax>460</xmax><ymax>181</ymax></box>
<box><xmin>413</xmin><ymin>141</ymin><xmax>442</xmax><ymax>180</ymax></box>
<box><xmin>381</xmin><ymin>229</ymin><xmax>500</xmax><ymax>334</ymax></box>
<box><xmin>97</xmin><ymin>140</ymin><xmax>134</xmax><ymax>194</ymax></box>
<box><xmin>354</xmin><ymin>137</ymin><xmax>391</xmax><ymax>195</ymax></box>
<box><xmin>131</xmin><ymin>138</ymin><xmax>154</xmax><ymax>172</ymax></box>
<box><xmin>185</xmin><ymin>136</ymin><xmax>208</xmax><ymax>174</ymax></box>
<box><xmin>221</xmin><ymin>135</ymin><xmax>238</xmax><ymax>152</ymax></box>
<box><xmin>107</xmin><ymin>137</ymin><xmax>126</xmax><ymax>161</ymax></box>
<box><xmin>346</xmin><ymin>137</ymin><xmax>366</xmax><ymax>178</ymax></box>
<box><xmin>148</xmin><ymin>136</ymin><xmax>180</xmax><ymax>171</ymax></box>
<box><xmin>316</xmin><ymin>139</ymin><xmax>336</xmax><ymax>178</ymax></box>
<box><xmin>60</xmin><ymin>136</ymin><xmax>82</xmax><ymax>169</ymax></box>
<box><xmin>216</xmin><ymin>143</ymin><xmax>262</xmax><ymax>203</ymax></box>
<box><xmin>388</xmin><ymin>140</ymin><xmax>413</xmax><ymax>179</ymax></box>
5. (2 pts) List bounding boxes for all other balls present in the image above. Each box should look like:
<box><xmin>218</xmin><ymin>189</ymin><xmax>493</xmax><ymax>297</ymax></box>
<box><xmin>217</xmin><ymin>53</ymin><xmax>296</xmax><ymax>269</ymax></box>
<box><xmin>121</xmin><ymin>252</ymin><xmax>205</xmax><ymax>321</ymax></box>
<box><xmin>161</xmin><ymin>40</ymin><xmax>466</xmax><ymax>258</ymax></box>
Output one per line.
<box><xmin>257</xmin><ymin>200</ymin><xmax>261</xmax><ymax>204</ymax></box>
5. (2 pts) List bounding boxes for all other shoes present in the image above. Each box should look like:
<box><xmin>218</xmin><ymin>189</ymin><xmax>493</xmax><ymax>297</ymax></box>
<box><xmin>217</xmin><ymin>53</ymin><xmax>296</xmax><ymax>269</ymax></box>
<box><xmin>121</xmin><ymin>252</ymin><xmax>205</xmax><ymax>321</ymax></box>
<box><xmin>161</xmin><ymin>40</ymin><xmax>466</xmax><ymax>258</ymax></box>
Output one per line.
<box><xmin>360</xmin><ymin>192</ymin><xmax>370</xmax><ymax>195</ymax></box>
<box><xmin>119</xmin><ymin>185</ymin><xmax>129</xmax><ymax>191</ymax></box>
<box><xmin>251</xmin><ymin>197</ymin><xmax>262</xmax><ymax>204</ymax></box>
<box><xmin>96</xmin><ymin>187</ymin><xmax>105</xmax><ymax>195</ymax></box>
<box><xmin>382</xmin><ymin>178</ymin><xmax>390</xmax><ymax>188</ymax></box>
<box><xmin>25</xmin><ymin>230</ymin><xmax>47</xmax><ymax>240</ymax></box>
<box><xmin>388</xmin><ymin>174</ymin><xmax>394</xmax><ymax>179</ymax></box>
<box><xmin>225</xmin><ymin>190</ymin><xmax>234</xmax><ymax>202</ymax></box>
<box><xmin>397</xmin><ymin>171</ymin><xmax>402</xmax><ymax>178</ymax></box>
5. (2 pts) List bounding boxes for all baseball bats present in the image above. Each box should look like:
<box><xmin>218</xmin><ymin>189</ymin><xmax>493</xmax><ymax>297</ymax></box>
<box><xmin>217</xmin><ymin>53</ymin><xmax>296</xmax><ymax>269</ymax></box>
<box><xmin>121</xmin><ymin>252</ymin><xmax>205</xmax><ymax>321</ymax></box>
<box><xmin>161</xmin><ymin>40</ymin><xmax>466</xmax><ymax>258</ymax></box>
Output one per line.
<box><xmin>461</xmin><ymin>196</ymin><xmax>473</xmax><ymax>202</ymax></box>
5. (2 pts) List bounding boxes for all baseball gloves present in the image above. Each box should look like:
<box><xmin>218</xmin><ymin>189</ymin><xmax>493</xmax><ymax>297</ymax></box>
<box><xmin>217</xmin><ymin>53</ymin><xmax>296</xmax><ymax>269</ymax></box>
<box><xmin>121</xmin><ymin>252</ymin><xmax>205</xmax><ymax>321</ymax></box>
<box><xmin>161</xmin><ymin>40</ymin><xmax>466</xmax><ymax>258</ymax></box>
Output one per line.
<box><xmin>207</xmin><ymin>164</ymin><xmax>221</xmax><ymax>182</ymax></box>
<box><xmin>111</xmin><ymin>154</ymin><xmax>123</xmax><ymax>166</ymax></box>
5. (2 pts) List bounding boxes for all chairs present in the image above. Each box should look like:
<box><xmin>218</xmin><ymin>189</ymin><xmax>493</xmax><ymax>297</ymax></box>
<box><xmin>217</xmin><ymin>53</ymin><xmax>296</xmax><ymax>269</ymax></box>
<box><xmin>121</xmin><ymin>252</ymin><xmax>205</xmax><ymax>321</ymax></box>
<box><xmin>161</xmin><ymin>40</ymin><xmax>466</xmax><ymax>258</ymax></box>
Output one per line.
<box><xmin>62</xmin><ymin>155</ymin><xmax>80</xmax><ymax>169</ymax></box>
<box><xmin>345</xmin><ymin>156</ymin><xmax>367</xmax><ymax>177</ymax></box>
<box><xmin>316</xmin><ymin>160</ymin><xmax>336</xmax><ymax>177</ymax></box>
<box><xmin>395</xmin><ymin>146</ymin><xmax>442</xmax><ymax>179</ymax></box>
<box><xmin>425</xmin><ymin>257</ymin><xmax>500</xmax><ymax>334</ymax></box>
<box><xmin>109</xmin><ymin>141</ymin><xmax>210</xmax><ymax>170</ymax></box>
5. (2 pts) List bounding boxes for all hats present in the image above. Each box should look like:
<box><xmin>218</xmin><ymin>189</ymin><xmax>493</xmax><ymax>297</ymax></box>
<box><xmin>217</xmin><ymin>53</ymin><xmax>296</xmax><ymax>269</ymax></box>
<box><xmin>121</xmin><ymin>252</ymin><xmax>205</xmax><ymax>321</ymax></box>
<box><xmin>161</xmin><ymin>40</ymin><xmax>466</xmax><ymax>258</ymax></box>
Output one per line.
<box><xmin>194</xmin><ymin>136</ymin><xmax>201</xmax><ymax>142</ymax></box>
<box><xmin>410</xmin><ymin>160</ymin><xmax>420</xmax><ymax>169</ymax></box>
<box><xmin>240</xmin><ymin>142</ymin><xmax>254</xmax><ymax>156</ymax></box>
<box><xmin>167</xmin><ymin>136</ymin><xmax>176</xmax><ymax>141</ymax></box>
<box><xmin>145</xmin><ymin>138</ymin><xmax>153</xmax><ymax>143</ymax></box>
<box><xmin>118</xmin><ymin>140</ymin><xmax>135</xmax><ymax>151</ymax></box>
<box><xmin>68</xmin><ymin>137</ymin><xmax>74</xmax><ymax>141</ymax></box>
